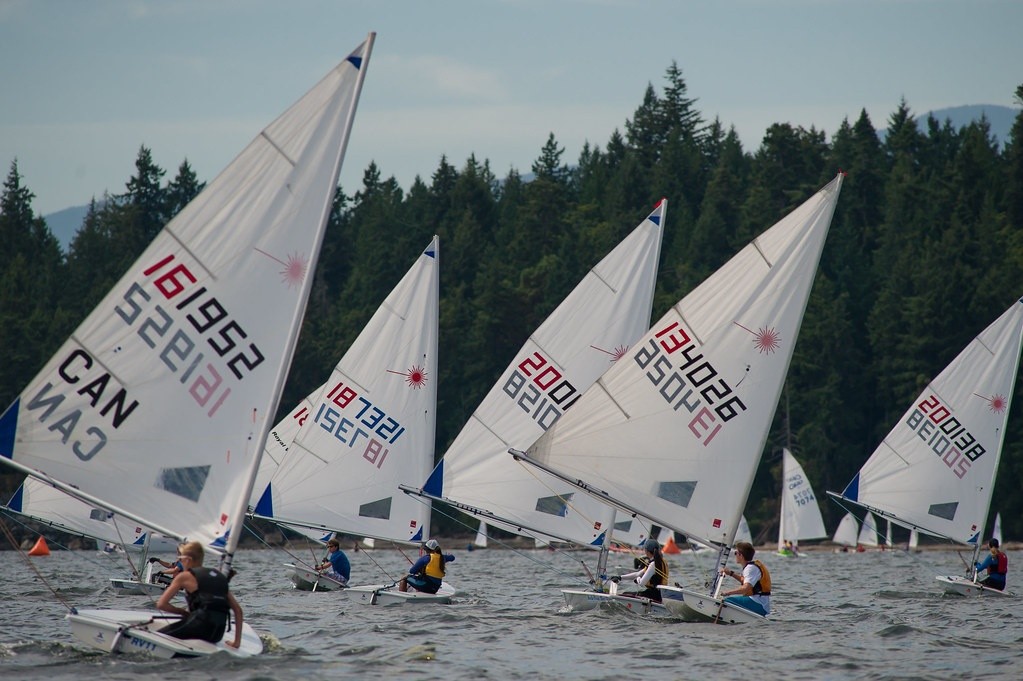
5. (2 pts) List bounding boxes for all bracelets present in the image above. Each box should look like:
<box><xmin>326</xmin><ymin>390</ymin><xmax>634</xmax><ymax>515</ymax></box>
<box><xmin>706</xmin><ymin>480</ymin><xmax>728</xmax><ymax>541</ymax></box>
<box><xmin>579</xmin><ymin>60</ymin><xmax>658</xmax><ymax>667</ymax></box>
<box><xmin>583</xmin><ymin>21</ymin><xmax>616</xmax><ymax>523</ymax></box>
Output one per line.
<box><xmin>321</xmin><ymin>565</ymin><xmax>323</xmax><ymax>570</ymax></box>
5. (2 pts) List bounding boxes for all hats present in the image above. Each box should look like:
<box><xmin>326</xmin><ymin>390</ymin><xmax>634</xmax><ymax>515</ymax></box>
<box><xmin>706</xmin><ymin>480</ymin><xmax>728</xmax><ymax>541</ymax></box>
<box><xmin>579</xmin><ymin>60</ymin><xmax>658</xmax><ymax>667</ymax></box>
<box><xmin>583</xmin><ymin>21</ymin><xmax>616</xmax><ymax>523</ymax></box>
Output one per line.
<box><xmin>426</xmin><ymin>539</ymin><xmax>440</xmax><ymax>550</ymax></box>
<box><xmin>643</xmin><ymin>539</ymin><xmax>660</xmax><ymax>552</ymax></box>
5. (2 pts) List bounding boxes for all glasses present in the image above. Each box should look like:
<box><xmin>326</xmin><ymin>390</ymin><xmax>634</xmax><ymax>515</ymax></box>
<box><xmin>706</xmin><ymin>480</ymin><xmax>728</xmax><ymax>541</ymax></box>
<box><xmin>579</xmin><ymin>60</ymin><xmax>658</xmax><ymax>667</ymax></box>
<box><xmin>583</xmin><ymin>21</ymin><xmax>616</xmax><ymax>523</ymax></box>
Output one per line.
<box><xmin>734</xmin><ymin>551</ymin><xmax>741</xmax><ymax>555</ymax></box>
<box><xmin>327</xmin><ymin>545</ymin><xmax>335</xmax><ymax>548</ymax></box>
<box><xmin>177</xmin><ymin>555</ymin><xmax>192</xmax><ymax>561</ymax></box>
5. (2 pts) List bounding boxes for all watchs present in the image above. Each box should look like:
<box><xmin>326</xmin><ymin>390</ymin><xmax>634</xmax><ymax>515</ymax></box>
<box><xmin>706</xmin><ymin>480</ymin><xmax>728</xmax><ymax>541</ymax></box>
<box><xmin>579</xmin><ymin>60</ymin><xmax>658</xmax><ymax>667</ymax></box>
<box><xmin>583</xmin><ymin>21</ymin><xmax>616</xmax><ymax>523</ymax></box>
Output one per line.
<box><xmin>730</xmin><ymin>571</ymin><xmax>734</xmax><ymax>576</ymax></box>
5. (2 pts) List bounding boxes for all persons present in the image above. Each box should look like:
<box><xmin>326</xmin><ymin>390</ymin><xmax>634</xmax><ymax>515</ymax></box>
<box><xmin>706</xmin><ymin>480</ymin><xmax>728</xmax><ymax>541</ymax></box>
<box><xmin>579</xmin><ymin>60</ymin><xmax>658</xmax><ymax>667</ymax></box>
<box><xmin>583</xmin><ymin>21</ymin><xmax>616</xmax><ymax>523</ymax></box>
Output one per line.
<box><xmin>609</xmin><ymin>539</ymin><xmax>669</xmax><ymax>604</ymax></box>
<box><xmin>855</xmin><ymin>544</ymin><xmax>866</xmax><ymax>553</ymax></box>
<box><xmin>399</xmin><ymin>540</ymin><xmax>455</xmax><ymax>593</ymax></box>
<box><xmin>719</xmin><ymin>541</ymin><xmax>772</xmax><ymax>617</ymax></box>
<box><xmin>973</xmin><ymin>539</ymin><xmax>1008</xmax><ymax>590</ymax></box>
<box><xmin>466</xmin><ymin>542</ymin><xmax>474</xmax><ymax>552</ymax></box>
<box><xmin>879</xmin><ymin>544</ymin><xmax>885</xmax><ymax>551</ymax></box>
<box><xmin>149</xmin><ymin>542</ymin><xmax>243</xmax><ymax>649</ymax></box>
<box><xmin>780</xmin><ymin>539</ymin><xmax>798</xmax><ymax>556</ymax></box>
<box><xmin>104</xmin><ymin>541</ymin><xmax>111</xmax><ymax>553</ymax></box>
<box><xmin>840</xmin><ymin>542</ymin><xmax>850</xmax><ymax>553</ymax></box>
<box><xmin>313</xmin><ymin>538</ymin><xmax>351</xmax><ymax>585</ymax></box>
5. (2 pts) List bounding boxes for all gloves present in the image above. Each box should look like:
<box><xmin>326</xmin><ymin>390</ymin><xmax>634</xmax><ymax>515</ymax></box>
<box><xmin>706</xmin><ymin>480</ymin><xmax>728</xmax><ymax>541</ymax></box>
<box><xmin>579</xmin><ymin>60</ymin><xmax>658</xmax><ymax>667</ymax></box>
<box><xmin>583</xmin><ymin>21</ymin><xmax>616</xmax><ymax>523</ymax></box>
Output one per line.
<box><xmin>610</xmin><ymin>575</ymin><xmax>621</xmax><ymax>583</ymax></box>
<box><xmin>150</xmin><ymin>557</ymin><xmax>160</xmax><ymax>564</ymax></box>
<box><xmin>157</xmin><ymin>571</ymin><xmax>164</xmax><ymax>576</ymax></box>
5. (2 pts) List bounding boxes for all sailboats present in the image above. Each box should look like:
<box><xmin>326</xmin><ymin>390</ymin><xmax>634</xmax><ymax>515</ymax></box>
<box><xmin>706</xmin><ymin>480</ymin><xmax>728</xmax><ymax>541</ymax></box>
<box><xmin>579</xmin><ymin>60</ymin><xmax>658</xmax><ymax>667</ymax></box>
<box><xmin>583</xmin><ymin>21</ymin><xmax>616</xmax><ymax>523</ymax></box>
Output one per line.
<box><xmin>988</xmin><ymin>513</ymin><xmax>1003</xmax><ymax>552</ymax></box>
<box><xmin>507</xmin><ymin>173</ymin><xmax>842</xmax><ymax>626</ymax></box>
<box><xmin>397</xmin><ymin>199</ymin><xmax>667</xmax><ymax>616</ymax></box>
<box><xmin>0</xmin><ymin>474</ymin><xmax>184</xmax><ymax>593</ymax></box>
<box><xmin>853</xmin><ymin>511</ymin><xmax>878</xmax><ymax>554</ymax></box>
<box><xmin>831</xmin><ymin>512</ymin><xmax>858</xmax><ymax>553</ymax></box>
<box><xmin>881</xmin><ymin>520</ymin><xmax>897</xmax><ymax>550</ymax></box>
<box><xmin>243</xmin><ymin>382</ymin><xmax>350</xmax><ymax>591</ymax></box>
<box><xmin>468</xmin><ymin>520</ymin><xmax>488</xmax><ymax>551</ymax></box>
<box><xmin>245</xmin><ymin>235</ymin><xmax>455</xmax><ymax>604</ymax></box>
<box><xmin>95</xmin><ymin>538</ymin><xmax>125</xmax><ymax>556</ymax></box>
<box><xmin>774</xmin><ymin>447</ymin><xmax>827</xmax><ymax>561</ymax></box>
<box><xmin>827</xmin><ymin>295</ymin><xmax>1023</xmax><ymax>597</ymax></box>
<box><xmin>906</xmin><ymin>529</ymin><xmax>923</xmax><ymax>553</ymax></box>
<box><xmin>683</xmin><ymin>516</ymin><xmax>754</xmax><ymax>554</ymax></box>
<box><xmin>533</xmin><ymin>538</ymin><xmax>555</xmax><ymax>553</ymax></box>
<box><xmin>612</xmin><ymin>512</ymin><xmax>652</xmax><ymax>554</ymax></box>
<box><xmin>0</xmin><ymin>24</ymin><xmax>381</xmax><ymax>660</ymax></box>
<box><xmin>658</xmin><ymin>526</ymin><xmax>680</xmax><ymax>555</ymax></box>
<box><xmin>351</xmin><ymin>537</ymin><xmax>378</xmax><ymax>552</ymax></box>
